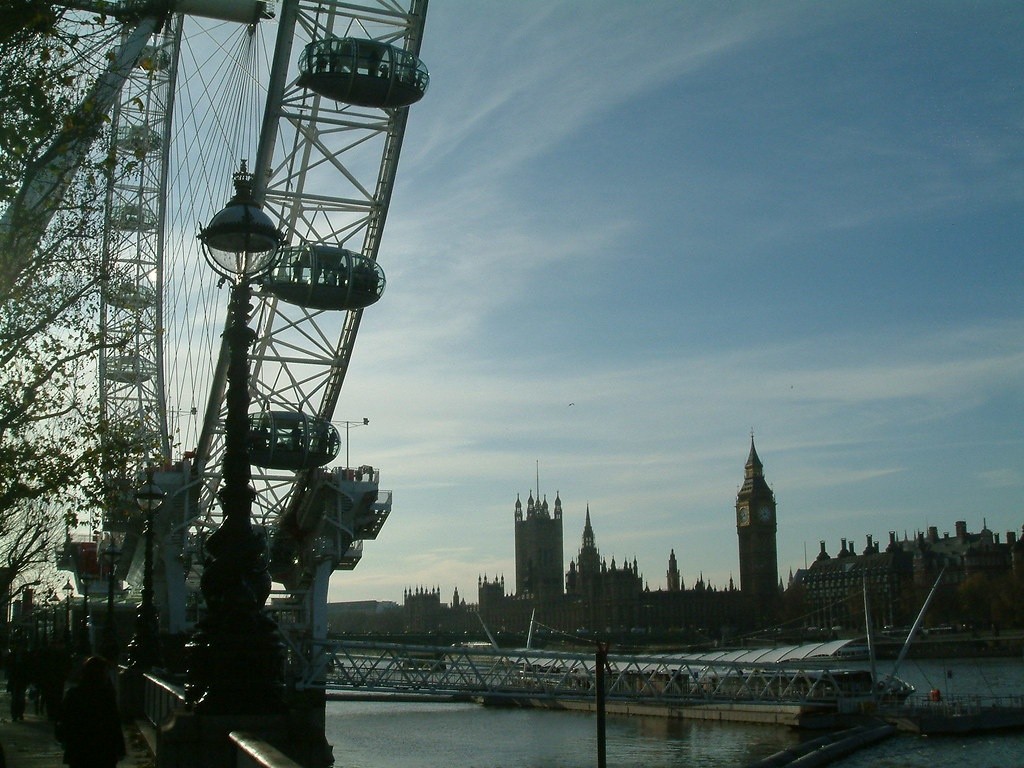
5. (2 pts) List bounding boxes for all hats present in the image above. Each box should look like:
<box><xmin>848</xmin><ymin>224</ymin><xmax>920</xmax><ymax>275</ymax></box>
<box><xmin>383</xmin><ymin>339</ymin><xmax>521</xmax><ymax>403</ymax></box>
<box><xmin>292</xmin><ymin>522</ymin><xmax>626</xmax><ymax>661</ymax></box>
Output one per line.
<box><xmin>80</xmin><ymin>655</ymin><xmax>106</xmax><ymax>671</ymax></box>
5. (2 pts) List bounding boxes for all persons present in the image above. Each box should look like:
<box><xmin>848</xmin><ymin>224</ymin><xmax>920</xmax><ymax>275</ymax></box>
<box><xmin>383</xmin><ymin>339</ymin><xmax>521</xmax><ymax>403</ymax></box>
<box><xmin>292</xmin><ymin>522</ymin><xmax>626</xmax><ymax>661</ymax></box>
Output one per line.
<box><xmin>5</xmin><ymin>643</ymin><xmax>70</xmax><ymax>722</ymax></box>
<box><xmin>290</xmin><ymin>253</ymin><xmax>379</xmax><ymax>295</ymax></box>
<box><xmin>252</xmin><ymin>426</ymin><xmax>337</xmax><ymax>456</ymax></box>
<box><xmin>53</xmin><ymin>656</ymin><xmax>126</xmax><ymax>768</ymax></box>
<box><xmin>315</xmin><ymin>47</ymin><xmax>423</xmax><ymax>90</ymax></box>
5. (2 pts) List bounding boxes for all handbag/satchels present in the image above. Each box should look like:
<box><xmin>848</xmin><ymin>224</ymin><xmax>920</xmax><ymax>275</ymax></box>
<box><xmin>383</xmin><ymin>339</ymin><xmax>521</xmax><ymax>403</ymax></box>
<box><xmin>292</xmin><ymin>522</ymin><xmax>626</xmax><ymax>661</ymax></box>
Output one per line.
<box><xmin>27</xmin><ymin>683</ymin><xmax>42</xmax><ymax>703</ymax></box>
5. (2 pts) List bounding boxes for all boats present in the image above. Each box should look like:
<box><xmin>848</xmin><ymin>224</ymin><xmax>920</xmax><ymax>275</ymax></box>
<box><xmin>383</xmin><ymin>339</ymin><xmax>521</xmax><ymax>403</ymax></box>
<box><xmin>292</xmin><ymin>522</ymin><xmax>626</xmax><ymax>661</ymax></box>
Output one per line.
<box><xmin>685</xmin><ymin>667</ymin><xmax>915</xmax><ymax>706</ymax></box>
<box><xmin>443</xmin><ymin>640</ymin><xmax>522</xmax><ymax>669</ymax></box>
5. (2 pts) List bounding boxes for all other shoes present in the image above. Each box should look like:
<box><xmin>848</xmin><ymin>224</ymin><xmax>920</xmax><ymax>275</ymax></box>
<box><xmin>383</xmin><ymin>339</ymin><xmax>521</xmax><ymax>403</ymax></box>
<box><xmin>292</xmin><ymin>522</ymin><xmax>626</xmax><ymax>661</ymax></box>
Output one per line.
<box><xmin>12</xmin><ymin>717</ymin><xmax>17</xmax><ymax>722</ymax></box>
<box><xmin>19</xmin><ymin>717</ymin><xmax>25</xmax><ymax>721</ymax></box>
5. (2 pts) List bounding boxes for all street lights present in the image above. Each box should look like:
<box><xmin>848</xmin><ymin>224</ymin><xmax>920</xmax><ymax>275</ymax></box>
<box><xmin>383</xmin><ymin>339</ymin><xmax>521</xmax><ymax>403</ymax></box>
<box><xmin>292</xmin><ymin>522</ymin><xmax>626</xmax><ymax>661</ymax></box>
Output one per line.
<box><xmin>35</xmin><ymin>602</ymin><xmax>40</xmax><ymax>646</ymax></box>
<box><xmin>49</xmin><ymin>593</ymin><xmax>60</xmax><ymax>644</ymax></box>
<box><xmin>63</xmin><ymin>579</ymin><xmax>74</xmax><ymax>648</ymax></box>
<box><xmin>129</xmin><ymin>470</ymin><xmax>164</xmax><ymax>665</ymax></box>
<box><xmin>100</xmin><ymin>537</ymin><xmax>122</xmax><ymax>660</ymax></box>
